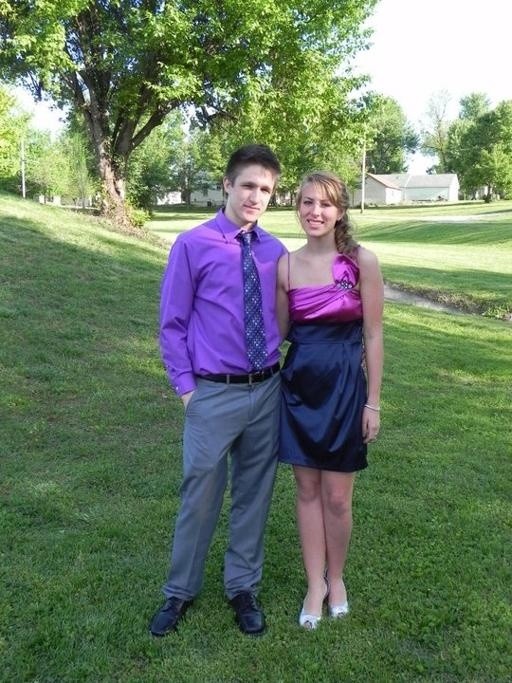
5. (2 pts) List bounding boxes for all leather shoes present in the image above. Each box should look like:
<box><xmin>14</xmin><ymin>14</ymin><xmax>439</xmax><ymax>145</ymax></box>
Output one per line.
<box><xmin>150</xmin><ymin>597</ymin><xmax>194</xmax><ymax>636</ymax></box>
<box><xmin>231</xmin><ymin>591</ymin><xmax>266</xmax><ymax>634</ymax></box>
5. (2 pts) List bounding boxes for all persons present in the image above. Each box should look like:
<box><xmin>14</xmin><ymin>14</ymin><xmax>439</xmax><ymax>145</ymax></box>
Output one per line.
<box><xmin>275</xmin><ymin>172</ymin><xmax>385</xmax><ymax>631</ymax></box>
<box><xmin>148</xmin><ymin>145</ymin><xmax>291</xmax><ymax>638</ymax></box>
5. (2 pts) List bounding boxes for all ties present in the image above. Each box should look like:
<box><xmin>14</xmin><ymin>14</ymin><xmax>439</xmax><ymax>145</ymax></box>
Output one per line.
<box><xmin>238</xmin><ymin>232</ymin><xmax>268</xmax><ymax>369</ymax></box>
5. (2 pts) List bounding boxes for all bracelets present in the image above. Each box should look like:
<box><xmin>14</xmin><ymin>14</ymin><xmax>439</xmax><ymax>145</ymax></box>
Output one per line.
<box><xmin>364</xmin><ymin>404</ymin><xmax>380</xmax><ymax>411</ymax></box>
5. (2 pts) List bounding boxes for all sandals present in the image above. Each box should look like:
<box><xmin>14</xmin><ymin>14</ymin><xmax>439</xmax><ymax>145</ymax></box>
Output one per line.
<box><xmin>298</xmin><ymin>569</ymin><xmax>350</xmax><ymax>630</ymax></box>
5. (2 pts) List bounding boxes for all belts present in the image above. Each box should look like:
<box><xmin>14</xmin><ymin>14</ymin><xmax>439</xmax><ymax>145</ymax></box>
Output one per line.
<box><xmin>200</xmin><ymin>362</ymin><xmax>280</xmax><ymax>384</ymax></box>
